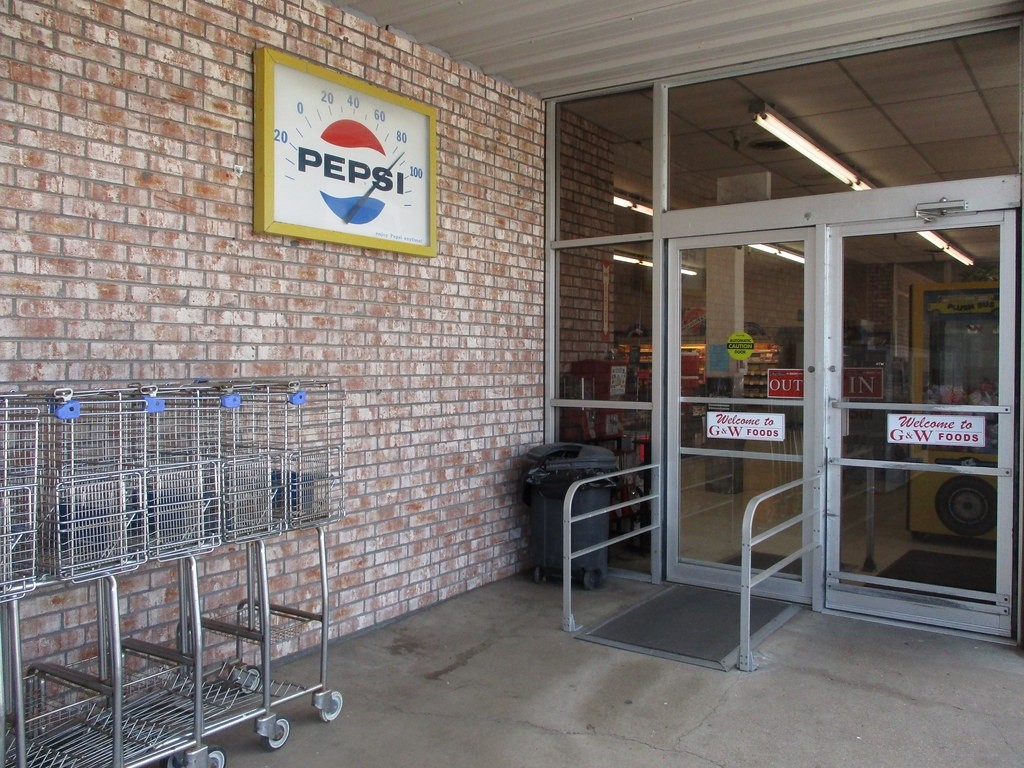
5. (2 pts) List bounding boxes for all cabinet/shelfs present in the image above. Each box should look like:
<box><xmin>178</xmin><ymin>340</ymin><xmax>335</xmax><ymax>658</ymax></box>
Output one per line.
<box><xmin>617</xmin><ymin>333</ymin><xmax>787</xmax><ymax>417</ymax></box>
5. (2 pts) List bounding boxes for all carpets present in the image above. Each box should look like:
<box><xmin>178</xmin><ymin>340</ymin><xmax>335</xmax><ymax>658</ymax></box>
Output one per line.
<box><xmin>865</xmin><ymin>548</ymin><xmax>999</xmax><ymax>606</ymax></box>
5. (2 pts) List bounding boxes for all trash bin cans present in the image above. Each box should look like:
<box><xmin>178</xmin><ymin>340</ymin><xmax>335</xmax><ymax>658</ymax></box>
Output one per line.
<box><xmin>525</xmin><ymin>443</ymin><xmax>619</xmax><ymax>591</ymax></box>
<box><xmin>705</xmin><ymin>436</ymin><xmax>748</xmax><ymax>495</ymax></box>
